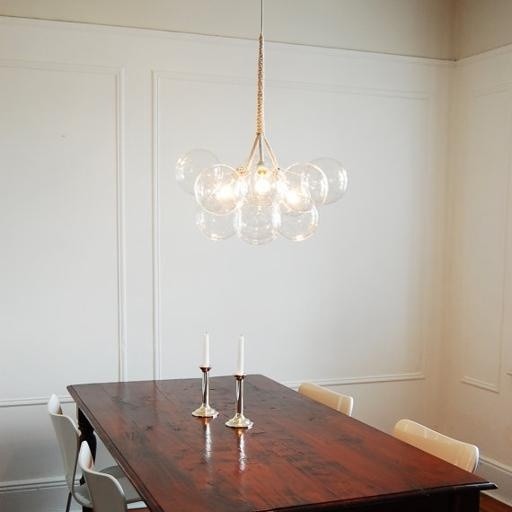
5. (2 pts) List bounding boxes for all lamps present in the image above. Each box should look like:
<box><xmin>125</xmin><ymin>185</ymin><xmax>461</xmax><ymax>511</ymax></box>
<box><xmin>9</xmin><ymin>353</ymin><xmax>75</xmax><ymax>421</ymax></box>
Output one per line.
<box><xmin>177</xmin><ymin>0</ymin><xmax>350</xmax><ymax>244</ymax></box>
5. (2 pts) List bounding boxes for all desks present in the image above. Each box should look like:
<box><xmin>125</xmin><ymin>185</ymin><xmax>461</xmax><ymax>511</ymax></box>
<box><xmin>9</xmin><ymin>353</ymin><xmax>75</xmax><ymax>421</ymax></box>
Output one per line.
<box><xmin>66</xmin><ymin>372</ymin><xmax>499</xmax><ymax>510</ymax></box>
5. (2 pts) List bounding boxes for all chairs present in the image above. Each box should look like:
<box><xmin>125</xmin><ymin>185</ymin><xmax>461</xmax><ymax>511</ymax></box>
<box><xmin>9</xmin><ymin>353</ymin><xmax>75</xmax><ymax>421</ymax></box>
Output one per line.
<box><xmin>391</xmin><ymin>419</ymin><xmax>479</xmax><ymax>474</ymax></box>
<box><xmin>299</xmin><ymin>382</ymin><xmax>354</xmax><ymax>416</ymax></box>
<box><xmin>46</xmin><ymin>392</ymin><xmax>136</xmax><ymax>509</ymax></box>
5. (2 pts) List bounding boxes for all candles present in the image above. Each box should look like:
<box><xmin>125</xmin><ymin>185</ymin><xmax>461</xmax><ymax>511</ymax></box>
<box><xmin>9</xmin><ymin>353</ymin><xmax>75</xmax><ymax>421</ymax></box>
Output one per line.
<box><xmin>203</xmin><ymin>332</ymin><xmax>213</xmax><ymax>367</ymax></box>
<box><xmin>236</xmin><ymin>336</ymin><xmax>244</xmax><ymax>374</ymax></box>
